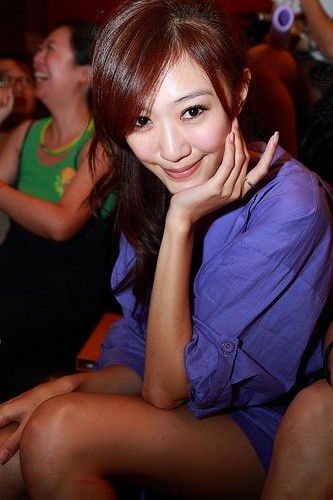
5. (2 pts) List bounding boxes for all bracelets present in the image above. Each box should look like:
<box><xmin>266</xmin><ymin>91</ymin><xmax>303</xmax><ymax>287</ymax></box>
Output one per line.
<box><xmin>322</xmin><ymin>339</ymin><xmax>332</xmax><ymax>387</ymax></box>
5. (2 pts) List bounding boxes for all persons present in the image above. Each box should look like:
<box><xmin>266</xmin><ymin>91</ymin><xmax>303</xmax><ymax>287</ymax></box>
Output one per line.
<box><xmin>0</xmin><ymin>16</ymin><xmax>126</xmax><ymax>399</ymax></box>
<box><xmin>237</xmin><ymin>0</ymin><xmax>332</xmax><ymax>190</ymax></box>
<box><xmin>255</xmin><ymin>317</ymin><xmax>333</xmax><ymax>500</ymax></box>
<box><xmin>0</xmin><ymin>0</ymin><xmax>333</xmax><ymax>500</ymax></box>
<box><xmin>0</xmin><ymin>49</ymin><xmax>35</xmax><ymax>129</ymax></box>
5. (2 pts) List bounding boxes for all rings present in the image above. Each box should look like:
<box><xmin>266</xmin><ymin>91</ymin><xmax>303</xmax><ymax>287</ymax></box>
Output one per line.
<box><xmin>244</xmin><ymin>177</ymin><xmax>256</xmax><ymax>189</ymax></box>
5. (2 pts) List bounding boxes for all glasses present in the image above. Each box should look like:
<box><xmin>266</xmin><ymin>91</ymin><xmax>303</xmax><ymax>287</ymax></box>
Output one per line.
<box><xmin>1</xmin><ymin>72</ymin><xmax>33</xmax><ymax>87</ymax></box>
